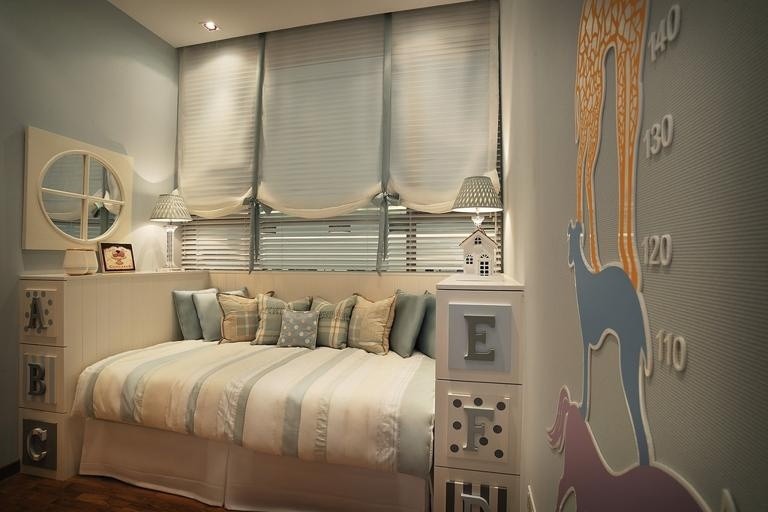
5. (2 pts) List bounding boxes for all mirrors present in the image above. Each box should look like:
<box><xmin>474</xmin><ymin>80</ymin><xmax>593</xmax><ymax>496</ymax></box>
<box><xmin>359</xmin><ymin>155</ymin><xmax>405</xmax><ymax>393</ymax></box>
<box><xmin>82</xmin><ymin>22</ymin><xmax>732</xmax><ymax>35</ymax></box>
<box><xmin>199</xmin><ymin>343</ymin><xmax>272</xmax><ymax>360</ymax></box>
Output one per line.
<box><xmin>38</xmin><ymin>149</ymin><xmax>126</xmax><ymax>244</ymax></box>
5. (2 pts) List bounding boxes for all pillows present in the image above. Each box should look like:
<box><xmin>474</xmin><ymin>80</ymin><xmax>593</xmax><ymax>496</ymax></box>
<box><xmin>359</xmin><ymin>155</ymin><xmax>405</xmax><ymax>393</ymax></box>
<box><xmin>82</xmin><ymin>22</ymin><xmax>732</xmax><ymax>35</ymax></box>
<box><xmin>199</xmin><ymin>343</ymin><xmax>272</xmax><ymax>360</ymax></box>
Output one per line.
<box><xmin>171</xmin><ymin>286</ymin><xmax>437</xmax><ymax>359</ymax></box>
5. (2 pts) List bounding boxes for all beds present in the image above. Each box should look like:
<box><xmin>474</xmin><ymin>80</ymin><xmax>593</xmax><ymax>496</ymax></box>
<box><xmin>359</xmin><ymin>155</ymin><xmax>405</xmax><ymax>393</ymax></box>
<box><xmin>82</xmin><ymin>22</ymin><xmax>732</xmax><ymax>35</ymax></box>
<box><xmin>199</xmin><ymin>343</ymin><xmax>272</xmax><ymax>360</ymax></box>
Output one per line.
<box><xmin>17</xmin><ymin>268</ymin><xmax>522</xmax><ymax>512</ymax></box>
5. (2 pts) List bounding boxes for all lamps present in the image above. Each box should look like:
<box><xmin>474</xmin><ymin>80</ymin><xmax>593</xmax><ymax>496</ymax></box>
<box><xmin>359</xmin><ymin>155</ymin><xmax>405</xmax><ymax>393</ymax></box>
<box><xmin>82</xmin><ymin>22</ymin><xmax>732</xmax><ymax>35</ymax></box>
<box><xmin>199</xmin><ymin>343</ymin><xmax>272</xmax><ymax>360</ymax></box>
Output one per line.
<box><xmin>451</xmin><ymin>176</ymin><xmax>504</xmax><ymax>228</ymax></box>
<box><xmin>151</xmin><ymin>193</ymin><xmax>194</xmax><ymax>271</ymax></box>
